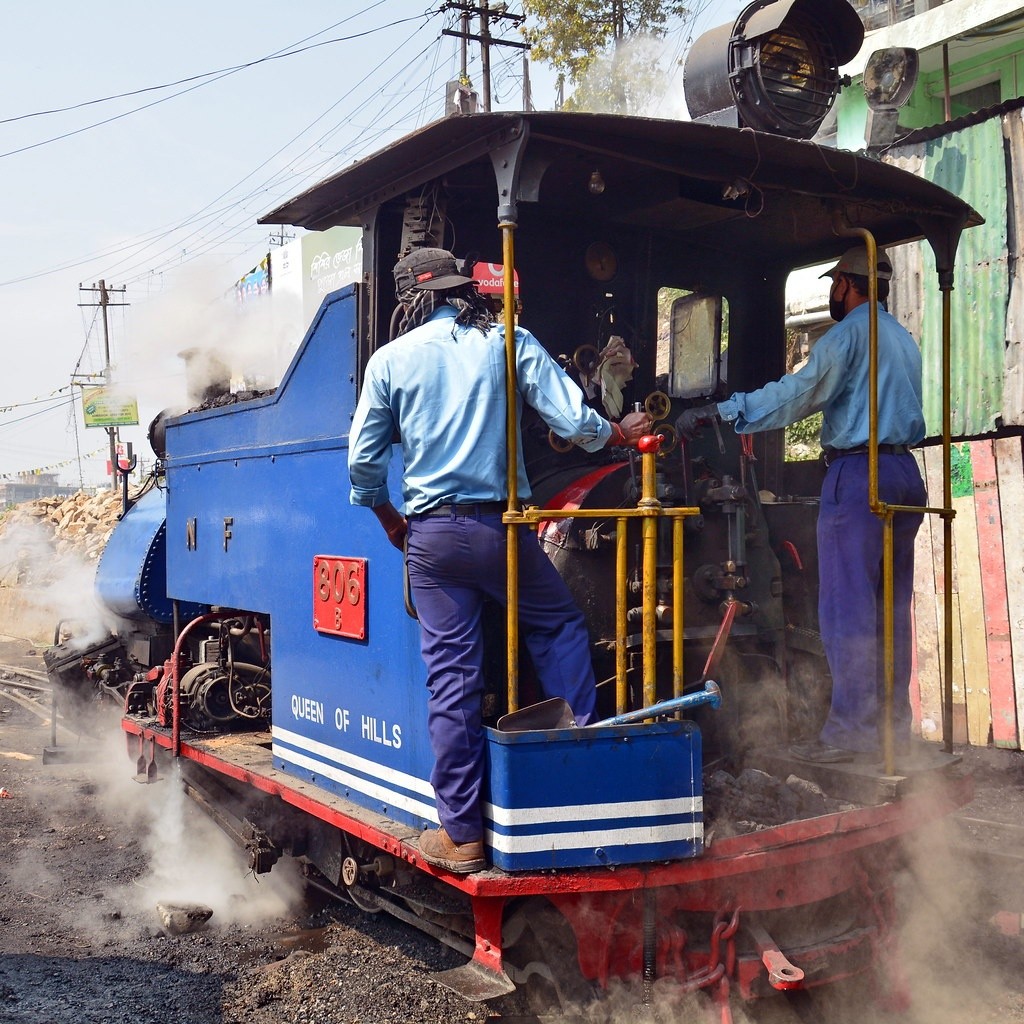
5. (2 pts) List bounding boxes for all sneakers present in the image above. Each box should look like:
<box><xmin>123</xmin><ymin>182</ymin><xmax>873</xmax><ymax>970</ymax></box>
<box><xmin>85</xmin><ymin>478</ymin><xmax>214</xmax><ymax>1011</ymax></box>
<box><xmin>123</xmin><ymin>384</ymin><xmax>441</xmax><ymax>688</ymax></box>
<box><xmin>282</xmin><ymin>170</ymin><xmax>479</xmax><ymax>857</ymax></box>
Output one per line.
<box><xmin>418</xmin><ymin>828</ymin><xmax>485</xmax><ymax>872</ymax></box>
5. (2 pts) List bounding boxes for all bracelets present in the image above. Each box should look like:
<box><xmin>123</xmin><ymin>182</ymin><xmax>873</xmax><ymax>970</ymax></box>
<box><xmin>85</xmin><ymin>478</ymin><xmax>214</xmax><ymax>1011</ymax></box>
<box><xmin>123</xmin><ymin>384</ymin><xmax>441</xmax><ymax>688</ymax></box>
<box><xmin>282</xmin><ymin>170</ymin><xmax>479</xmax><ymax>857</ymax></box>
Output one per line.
<box><xmin>609</xmin><ymin>421</ymin><xmax>626</xmax><ymax>446</ymax></box>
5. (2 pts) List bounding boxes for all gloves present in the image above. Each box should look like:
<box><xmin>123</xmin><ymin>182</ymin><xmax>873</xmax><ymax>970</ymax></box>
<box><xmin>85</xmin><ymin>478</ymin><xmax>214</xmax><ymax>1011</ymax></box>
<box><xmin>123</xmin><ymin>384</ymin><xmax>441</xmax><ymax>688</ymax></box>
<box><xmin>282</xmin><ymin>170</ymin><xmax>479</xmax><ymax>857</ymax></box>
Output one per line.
<box><xmin>676</xmin><ymin>404</ymin><xmax>722</xmax><ymax>440</ymax></box>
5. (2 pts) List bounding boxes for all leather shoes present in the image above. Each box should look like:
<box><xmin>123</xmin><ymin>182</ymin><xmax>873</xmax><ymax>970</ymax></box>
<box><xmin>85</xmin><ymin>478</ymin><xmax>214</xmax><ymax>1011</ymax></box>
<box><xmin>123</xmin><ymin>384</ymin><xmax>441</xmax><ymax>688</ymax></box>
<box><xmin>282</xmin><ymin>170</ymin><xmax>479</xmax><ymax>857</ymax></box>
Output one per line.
<box><xmin>888</xmin><ymin>738</ymin><xmax>912</xmax><ymax>757</ymax></box>
<box><xmin>791</xmin><ymin>735</ymin><xmax>880</xmax><ymax>765</ymax></box>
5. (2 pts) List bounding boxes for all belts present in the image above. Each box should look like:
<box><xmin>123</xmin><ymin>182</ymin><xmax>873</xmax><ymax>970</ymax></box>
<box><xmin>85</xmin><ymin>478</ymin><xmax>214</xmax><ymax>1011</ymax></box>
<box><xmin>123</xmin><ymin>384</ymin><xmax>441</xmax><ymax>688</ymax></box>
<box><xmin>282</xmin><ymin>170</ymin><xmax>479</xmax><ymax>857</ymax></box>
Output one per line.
<box><xmin>419</xmin><ymin>501</ymin><xmax>522</xmax><ymax>517</ymax></box>
<box><xmin>824</xmin><ymin>444</ymin><xmax>910</xmax><ymax>467</ymax></box>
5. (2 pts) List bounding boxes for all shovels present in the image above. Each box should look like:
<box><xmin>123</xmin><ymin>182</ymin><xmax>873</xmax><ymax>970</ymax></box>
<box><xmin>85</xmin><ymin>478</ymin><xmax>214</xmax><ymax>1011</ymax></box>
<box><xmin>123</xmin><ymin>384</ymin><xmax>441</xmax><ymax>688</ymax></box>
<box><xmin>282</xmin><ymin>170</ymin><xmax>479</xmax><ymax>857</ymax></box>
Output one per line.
<box><xmin>497</xmin><ymin>680</ymin><xmax>722</xmax><ymax>732</ymax></box>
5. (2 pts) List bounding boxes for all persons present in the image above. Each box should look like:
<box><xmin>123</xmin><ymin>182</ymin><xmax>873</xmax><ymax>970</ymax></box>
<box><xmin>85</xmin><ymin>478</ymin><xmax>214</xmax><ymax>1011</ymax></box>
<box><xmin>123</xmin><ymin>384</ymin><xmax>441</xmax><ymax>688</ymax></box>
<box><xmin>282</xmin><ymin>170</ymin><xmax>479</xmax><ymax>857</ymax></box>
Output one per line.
<box><xmin>677</xmin><ymin>244</ymin><xmax>929</xmax><ymax>763</ymax></box>
<box><xmin>349</xmin><ymin>248</ymin><xmax>652</xmax><ymax>873</ymax></box>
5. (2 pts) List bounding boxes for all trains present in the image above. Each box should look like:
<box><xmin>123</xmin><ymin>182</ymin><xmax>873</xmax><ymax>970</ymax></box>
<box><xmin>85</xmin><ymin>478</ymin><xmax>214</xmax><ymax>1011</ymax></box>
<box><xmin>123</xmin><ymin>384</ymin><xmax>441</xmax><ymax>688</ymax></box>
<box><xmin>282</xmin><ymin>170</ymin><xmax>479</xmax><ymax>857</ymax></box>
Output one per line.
<box><xmin>38</xmin><ymin>2</ymin><xmax>989</xmax><ymax>1024</ymax></box>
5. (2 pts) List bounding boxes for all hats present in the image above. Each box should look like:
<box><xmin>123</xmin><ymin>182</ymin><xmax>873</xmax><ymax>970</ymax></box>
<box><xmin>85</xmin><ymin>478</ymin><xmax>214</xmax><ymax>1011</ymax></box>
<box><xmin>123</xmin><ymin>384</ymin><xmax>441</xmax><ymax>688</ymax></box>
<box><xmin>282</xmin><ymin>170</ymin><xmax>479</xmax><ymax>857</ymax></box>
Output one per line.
<box><xmin>818</xmin><ymin>246</ymin><xmax>892</xmax><ymax>280</ymax></box>
<box><xmin>394</xmin><ymin>248</ymin><xmax>480</xmax><ymax>295</ymax></box>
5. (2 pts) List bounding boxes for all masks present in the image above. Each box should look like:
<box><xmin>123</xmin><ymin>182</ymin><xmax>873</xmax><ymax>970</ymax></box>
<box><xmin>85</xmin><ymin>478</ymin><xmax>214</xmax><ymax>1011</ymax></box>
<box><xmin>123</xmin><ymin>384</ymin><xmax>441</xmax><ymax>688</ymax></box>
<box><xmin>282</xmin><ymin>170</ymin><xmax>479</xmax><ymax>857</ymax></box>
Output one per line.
<box><xmin>829</xmin><ymin>280</ymin><xmax>848</xmax><ymax>322</ymax></box>
